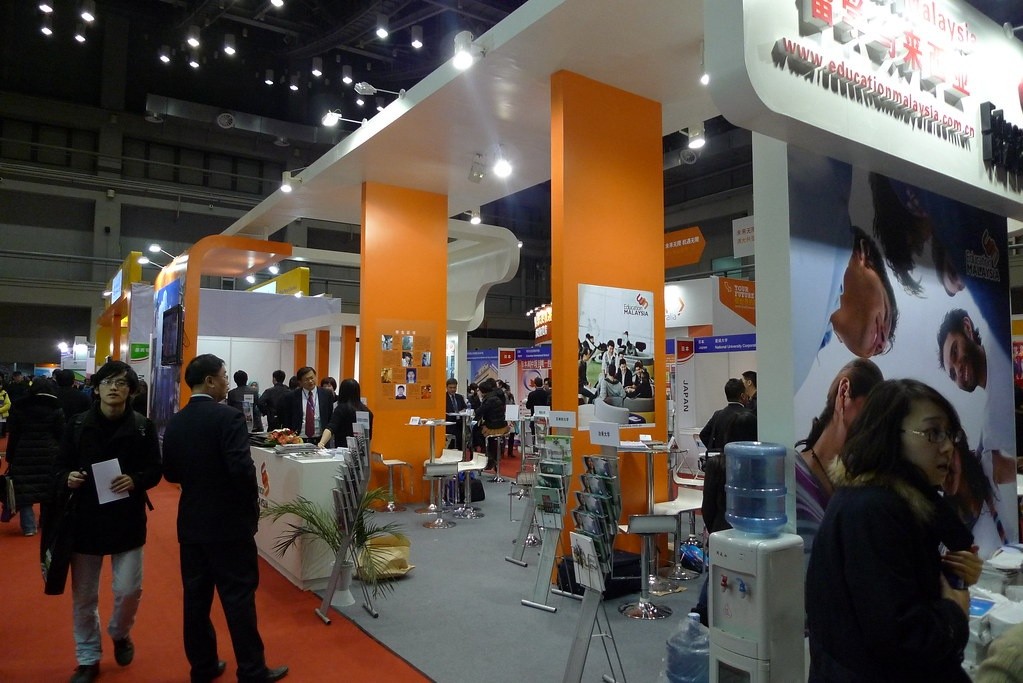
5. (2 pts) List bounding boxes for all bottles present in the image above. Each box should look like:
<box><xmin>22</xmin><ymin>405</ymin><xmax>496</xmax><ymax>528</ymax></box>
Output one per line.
<box><xmin>723</xmin><ymin>441</ymin><xmax>787</xmax><ymax>535</ymax></box>
<box><xmin>467</xmin><ymin>402</ymin><xmax>471</xmax><ymax>415</ymax></box>
<box><xmin>666</xmin><ymin>613</ymin><xmax>709</xmax><ymax>683</ymax></box>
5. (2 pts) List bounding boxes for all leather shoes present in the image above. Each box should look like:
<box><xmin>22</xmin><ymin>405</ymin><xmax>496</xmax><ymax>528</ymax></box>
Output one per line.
<box><xmin>215</xmin><ymin>662</ymin><xmax>226</xmax><ymax>679</ymax></box>
<box><xmin>262</xmin><ymin>666</ymin><xmax>288</xmax><ymax>683</ymax></box>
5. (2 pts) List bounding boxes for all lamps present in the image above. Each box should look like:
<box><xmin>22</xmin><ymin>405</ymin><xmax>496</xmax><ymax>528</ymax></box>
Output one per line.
<box><xmin>678</xmin><ymin>122</ymin><xmax>705</xmax><ymax>164</ymax></box>
<box><xmin>157</xmin><ymin>0</ymin><xmax>487</xmax><ymax>192</ymax></box>
<box><xmin>149</xmin><ymin>243</ymin><xmax>175</xmax><ymax>259</ymax></box>
<box><xmin>103</xmin><ymin>290</ymin><xmax>120</xmax><ymax>298</ymax></box>
<box><xmin>37</xmin><ymin>0</ymin><xmax>96</xmax><ymax>43</ymax></box>
<box><xmin>138</xmin><ymin>256</ymin><xmax>163</xmax><ymax>269</ymax></box>
<box><xmin>525</xmin><ymin>303</ymin><xmax>551</xmax><ymax>317</ymax></box>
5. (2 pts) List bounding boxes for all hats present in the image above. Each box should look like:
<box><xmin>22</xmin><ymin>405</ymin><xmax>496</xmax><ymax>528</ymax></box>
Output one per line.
<box><xmin>12</xmin><ymin>371</ymin><xmax>22</xmax><ymax>375</ymax></box>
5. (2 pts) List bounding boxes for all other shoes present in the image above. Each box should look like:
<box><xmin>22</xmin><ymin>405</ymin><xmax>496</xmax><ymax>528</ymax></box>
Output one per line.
<box><xmin>114</xmin><ymin>635</ymin><xmax>134</xmax><ymax>667</ymax></box>
<box><xmin>24</xmin><ymin>523</ymin><xmax>37</xmax><ymax>536</ymax></box>
<box><xmin>508</xmin><ymin>452</ymin><xmax>516</xmax><ymax>458</ymax></box>
<box><xmin>70</xmin><ymin>662</ymin><xmax>99</xmax><ymax>683</ymax></box>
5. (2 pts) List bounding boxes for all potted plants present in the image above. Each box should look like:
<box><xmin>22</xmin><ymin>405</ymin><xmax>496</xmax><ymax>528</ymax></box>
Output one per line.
<box><xmin>258</xmin><ymin>483</ymin><xmax>408</xmax><ymax>591</ymax></box>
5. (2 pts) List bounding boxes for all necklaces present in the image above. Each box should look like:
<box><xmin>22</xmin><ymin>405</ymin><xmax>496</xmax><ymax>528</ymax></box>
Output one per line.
<box><xmin>809</xmin><ymin>448</ymin><xmax>835</xmax><ymax>495</ymax></box>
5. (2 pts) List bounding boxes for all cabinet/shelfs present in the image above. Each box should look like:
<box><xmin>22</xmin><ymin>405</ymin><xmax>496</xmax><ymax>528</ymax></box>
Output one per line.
<box><xmin>315</xmin><ymin>411</ymin><xmax>379</xmax><ymax>625</ymax></box>
<box><xmin>502</xmin><ymin>406</ymin><xmax>628</xmax><ymax>683</ymax></box>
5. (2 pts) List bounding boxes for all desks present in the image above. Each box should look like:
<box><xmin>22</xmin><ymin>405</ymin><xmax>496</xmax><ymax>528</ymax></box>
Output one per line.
<box><xmin>249</xmin><ymin>445</ymin><xmax>361</xmax><ymax>592</ymax></box>
<box><xmin>404</xmin><ymin>421</ymin><xmax>457</xmax><ymax>515</ymax></box>
<box><xmin>616</xmin><ymin>447</ymin><xmax>688</xmax><ymax>620</ymax></box>
<box><xmin>446</xmin><ymin>412</ymin><xmax>476</xmax><ymax>451</ymax></box>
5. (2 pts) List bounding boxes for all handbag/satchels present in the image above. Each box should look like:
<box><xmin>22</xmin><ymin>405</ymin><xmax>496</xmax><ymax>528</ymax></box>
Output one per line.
<box><xmin>445</xmin><ymin>479</ymin><xmax>486</xmax><ymax>505</ymax></box>
<box><xmin>41</xmin><ymin>491</ymin><xmax>73</xmax><ymax>595</ymax></box>
<box><xmin>0</xmin><ymin>465</ymin><xmax>18</xmax><ymax>522</ymax></box>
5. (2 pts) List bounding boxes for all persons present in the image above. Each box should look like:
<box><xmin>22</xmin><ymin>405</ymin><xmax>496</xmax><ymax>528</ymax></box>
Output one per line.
<box><xmin>0</xmin><ymin>369</ymin><xmax>149</xmax><ymax>537</ymax></box>
<box><xmin>690</xmin><ymin>371</ymin><xmax>767</xmax><ymax>628</ymax></box>
<box><xmin>446</xmin><ymin>376</ymin><xmax>552</xmax><ymax>472</ymax></box>
<box><xmin>382</xmin><ymin>335</ymin><xmax>432</xmax><ymax>399</ymax></box>
<box><xmin>161</xmin><ymin>355</ymin><xmax>290</xmax><ymax>683</ymax></box>
<box><xmin>49</xmin><ymin>360</ymin><xmax>162</xmax><ymax>683</ymax></box>
<box><xmin>805</xmin><ymin>379</ymin><xmax>983</xmax><ymax>683</ymax></box>
<box><xmin>578</xmin><ymin>331</ymin><xmax>653</xmax><ymax>406</ymax></box>
<box><xmin>789</xmin><ymin>163</ymin><xmax>1017</xmax><ymax>558</ymax></box>
<box><xmin>228</xmin><ymin>367</ymin><xmax>375</xmax><ymax>548</ymax></box>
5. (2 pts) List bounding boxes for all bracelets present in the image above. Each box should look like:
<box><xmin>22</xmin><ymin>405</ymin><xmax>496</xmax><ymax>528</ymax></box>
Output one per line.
<box><xmin>319</xmin><ymin>441</ymin><xmax>325</xmax><ymax>445</ymax></box>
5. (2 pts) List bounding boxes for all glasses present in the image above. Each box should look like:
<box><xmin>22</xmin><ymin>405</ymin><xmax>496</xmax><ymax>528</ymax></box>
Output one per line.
<box><xmin>100</xmin><ymin>379</ymin><xmax>128</xmax><ymax>387</ymax></box>
<box><xmin>215</xmin><ymin>375</ymin><xmax>228</xmax><ymax>381</ymax></box>
<box><xmin>904</xmin><ymin>428</ymin><xmax>962</xmax><ymax>444</ymax></box>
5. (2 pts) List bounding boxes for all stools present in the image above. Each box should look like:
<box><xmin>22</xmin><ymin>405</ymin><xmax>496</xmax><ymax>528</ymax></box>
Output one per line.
<box><xmin>371</xmin><ymin>450</ymin><xmax>414</xmax><ymax>513</ymax></box>
<box><xmin>485</xmin><ymin>431</ymin><xmax>511</xmax><ymax>483</ymax></box>
<box><xmin>618</xmin><ymin>434</ymin><xmax>725</xmax><ymax>620</ymax></box>
<box><xmin>422</xmin><ymin>449</ymin><xmax>489</xmax><ymax>529</ymax></box>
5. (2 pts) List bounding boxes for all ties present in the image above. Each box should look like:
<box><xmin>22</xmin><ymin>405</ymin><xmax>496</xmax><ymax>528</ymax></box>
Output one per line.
<box><xmin>452</xmin><ymin>395</ymin><xmax>457</xmax><ymax>411</ymax></box>
<box><xmin>305</xmin><ymin>391</ymin><xmax>315</xmax><ymax>438</ymax></box>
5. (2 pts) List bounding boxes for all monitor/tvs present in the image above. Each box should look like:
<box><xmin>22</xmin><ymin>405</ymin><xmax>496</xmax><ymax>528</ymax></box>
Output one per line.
<box><xmin>161</xmin><ymin>304</ymin><xmax>184</xmax><ymax>366</ymax></box>
